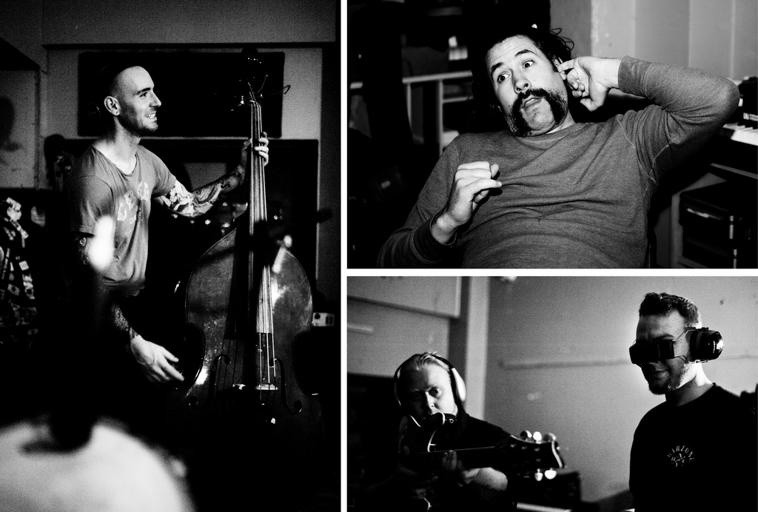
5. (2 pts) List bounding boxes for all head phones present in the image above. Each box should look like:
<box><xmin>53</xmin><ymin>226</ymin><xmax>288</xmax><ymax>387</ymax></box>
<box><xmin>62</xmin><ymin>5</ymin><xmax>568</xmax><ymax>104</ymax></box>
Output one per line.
<box><xmin>393</xmin><ymin>352</ymin><xmax>468</xmax><ymax>408</ymax></box>
<box><xmin>628</xmin><ymin>326</ymin><xmax>724</xmax><ymax>366</ymax></box>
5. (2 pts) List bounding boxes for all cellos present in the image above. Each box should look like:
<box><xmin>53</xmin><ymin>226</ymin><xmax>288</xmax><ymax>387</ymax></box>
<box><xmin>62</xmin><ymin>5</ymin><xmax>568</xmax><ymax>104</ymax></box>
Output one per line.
<box><xmin>158</xmin><ymin>45</ymin><xmax>340</xmax><ymax>512</ymax></box>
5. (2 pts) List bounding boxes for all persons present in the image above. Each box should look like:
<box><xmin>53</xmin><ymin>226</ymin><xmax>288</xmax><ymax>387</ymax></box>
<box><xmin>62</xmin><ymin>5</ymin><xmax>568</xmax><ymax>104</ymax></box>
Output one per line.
<box><xmin>630</xmin><ymin>292</ymin><xmax>757</xmax><ymax>510</ymax></box>
<box><xmin>62</xmin><ymin>66</ymin><xmax>270</xmax><ymax>384</ymax></box>
<box><xmin>377</xmin><ymin>25</ymin><xmax>740</xmax><ymax>268</ymax></box>
<box><xmin>393</xmin><ymin>354</ymin><xmax>515</xmax><ymax>493</ymax></box>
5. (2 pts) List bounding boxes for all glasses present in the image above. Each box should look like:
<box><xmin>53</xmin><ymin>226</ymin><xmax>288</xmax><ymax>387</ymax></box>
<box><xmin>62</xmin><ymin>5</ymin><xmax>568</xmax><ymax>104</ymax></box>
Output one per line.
<box><xmin>630</xmin><ymin>327</ymin><xmax>688</xmax><ymax>364</ymax></box>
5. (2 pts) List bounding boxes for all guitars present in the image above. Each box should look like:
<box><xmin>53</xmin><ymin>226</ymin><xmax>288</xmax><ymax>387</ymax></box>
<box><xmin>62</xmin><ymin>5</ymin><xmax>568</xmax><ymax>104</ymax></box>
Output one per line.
<box><xmin>348</xmin><ymin>416</ymin><xmax>565</xmax><ymax>509</ymax></box>
